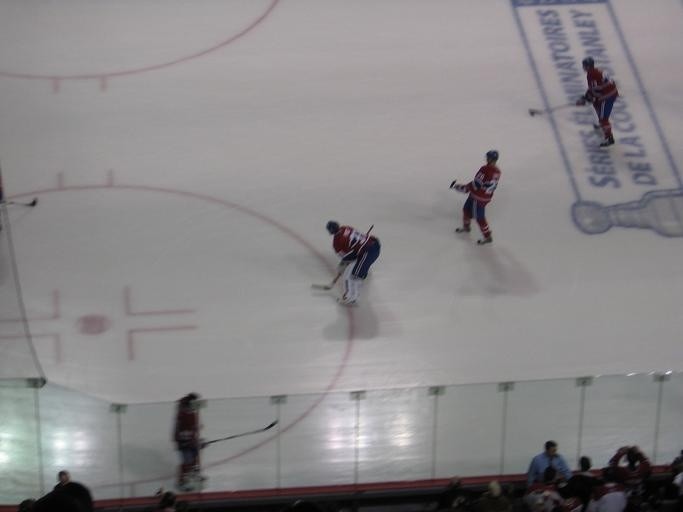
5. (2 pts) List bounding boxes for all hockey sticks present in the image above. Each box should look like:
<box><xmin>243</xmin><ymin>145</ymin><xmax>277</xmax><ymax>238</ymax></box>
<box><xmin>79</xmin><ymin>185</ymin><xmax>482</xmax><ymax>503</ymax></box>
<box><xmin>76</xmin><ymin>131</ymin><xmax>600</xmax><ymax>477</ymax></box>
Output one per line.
<box><xmin>0</xmin><ymin>197</ymin><xmax>37</xmax><ymax>207</ymax></box>
<box><xmin>202</xmin><ymin>418</ymin><xmax>278</xmax><ymax>444</ymax></box>
<box><xmin>311</xmin><ymin>225</ymin><xmax>374</xmax><ymax>290</ymax></box>
<box><xmin>527</xmin><ymin>101</ymin><xmax>580</xmax><ymax>116</ymax></box>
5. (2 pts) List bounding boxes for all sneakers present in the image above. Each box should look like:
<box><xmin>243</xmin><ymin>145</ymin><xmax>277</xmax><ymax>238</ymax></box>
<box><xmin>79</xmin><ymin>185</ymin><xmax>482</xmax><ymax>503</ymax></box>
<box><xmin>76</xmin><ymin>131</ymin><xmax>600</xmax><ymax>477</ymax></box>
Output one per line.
<box><xmin>477</xmin><ymin>237</ymin><xmax>493</xmax><ymax>245</ymax></box>
<box><xmin>600</xmin><ymin>134</ymin><xmax>615</xmax><ymax>147</ymax></box>
<box><xmin>455</xmin><ymin>227</ymin><xmax>471</xmax><ymax>233</ymax></box>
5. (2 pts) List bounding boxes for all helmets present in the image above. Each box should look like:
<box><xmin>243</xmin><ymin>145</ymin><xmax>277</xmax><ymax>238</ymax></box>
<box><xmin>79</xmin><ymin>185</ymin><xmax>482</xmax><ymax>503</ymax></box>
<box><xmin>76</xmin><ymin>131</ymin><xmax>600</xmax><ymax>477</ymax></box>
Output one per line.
<box><xmin>486</xmin><ymin>150</ymin><xmax>499</xmax><ymax>161</ymax></box>
<box><xmin>326</xmin><ymin>220</ymin><xmax>339</xmax><ymax>234</ymax></box>
<box><xmin>582</xmin><ymin>56</ymin><xmax>595</xmax><ymax>67</ymax></box>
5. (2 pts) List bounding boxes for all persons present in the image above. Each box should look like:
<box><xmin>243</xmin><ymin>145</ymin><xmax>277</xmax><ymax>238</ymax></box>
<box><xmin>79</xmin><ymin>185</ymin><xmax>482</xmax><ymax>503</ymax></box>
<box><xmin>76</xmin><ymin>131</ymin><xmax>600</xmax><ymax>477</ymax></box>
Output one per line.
<box><xmin>326</xmin><ymin>220</ymin><xmax>380</xmax><ymax>306</ymax></box>
<box><xmin>454</xmin><ymin>150</ymin><xmax>502</xmax><ymax>244</ymax></box>
<box><xmin>20</xmin><ymin>441</ymin><xmax>189</xmax><ymax>511</ymax></box>
<box><xmin>439</xmin><ymin>441</ymin><xmax>683</xmax><ymax>511</ymax></box>
<box><xmin>175</xmin><ymin>393</ymin><xmax>209</xmax><ymax>487</ymax></box>
<box><xmin>577</xmin><ymin>58</ymin><xmax>619</xmax><ymax>146</ymax></box>
